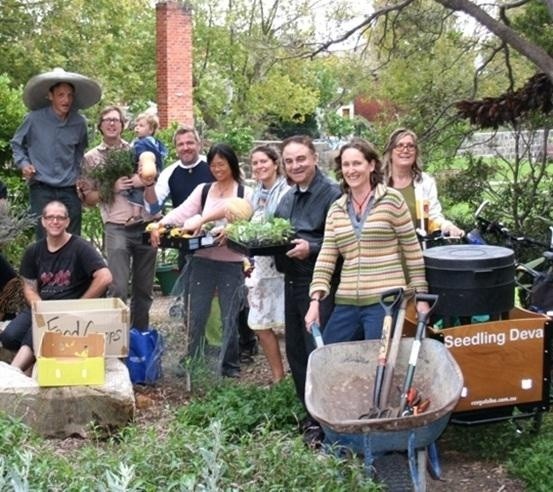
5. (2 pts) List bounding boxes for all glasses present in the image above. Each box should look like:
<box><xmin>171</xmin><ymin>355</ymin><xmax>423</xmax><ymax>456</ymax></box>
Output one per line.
<box><xmin>43</xmin><ymin>216</ymin><xmax>67</xmax><ymax>221</ymax></box>
<box><xmin>101</xmin><ymin>119</ymin><xmax>121</xmax><ymax>123</ymax></box>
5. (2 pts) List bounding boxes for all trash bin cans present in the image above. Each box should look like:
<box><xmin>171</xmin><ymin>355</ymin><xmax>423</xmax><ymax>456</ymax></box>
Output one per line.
<box><xmin>422</xmin><ymin>243</ymin><xmax>516</xmax><ymax>425</ymax></box>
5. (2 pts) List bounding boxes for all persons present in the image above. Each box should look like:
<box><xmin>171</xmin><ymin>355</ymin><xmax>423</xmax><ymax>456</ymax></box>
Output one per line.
<box><xmin>83</xmin><ymin>106</ymin><xmax>162</xmax><ymax>332</ymax></box>
<box><xmin>381</xmin><ymin>128</ymin><xmax>465</xmax><ymax>250</ymax></box>
<box><xmin>213</xmin><ymin>146</ymin><xmax>291</xmax><ymax>389</ymax></box>
<box><xmin>150</xmin><ymin>143</ymin><xmax>254</xmax><ymax>377</ymax></box>
<box><xmin>137</xmin><ymin>126</ymin><xmax>259</xmax><ymax>363</ymax></box>
<box><xmin>124</xmin><ymin>113</ymin><xmax>168</xmax><ymax>227</ymax></box>
<box><xmin>304</xmin><ymin>137</ymin><xmax>428</xmax><ymax>458</ymax></box>
<box><xmin>270</xmin><ymin>134</ymin><xmax>344</xmax><ymax>434</ymax></box>
<box><xmin>0</xmin><ymin>200</ymin><xmax>114</xmax><ymax>371</ymax></box>
<box><xmin>8</xmin><ymin>67</ymin><xmax>102</xmax><ymax>241</ymax></box>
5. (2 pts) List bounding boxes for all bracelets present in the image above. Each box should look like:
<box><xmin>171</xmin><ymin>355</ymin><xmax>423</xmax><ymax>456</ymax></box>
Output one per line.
<box><xmin>308</xmin><ymin>298</ymin><xmax>322</xmax><ymax>304</ymax></box>
<box><xmin>146</xmin><ymin>183</ymin><xmax>154</xmax><ymax>187</ymax></box>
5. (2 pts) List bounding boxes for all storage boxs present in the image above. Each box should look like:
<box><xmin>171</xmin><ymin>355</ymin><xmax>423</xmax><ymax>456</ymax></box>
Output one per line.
<box><xmin>35</xmin><ymin>327</ymin><xmax>106</xmax><ymax>386</ymax></box>
<box><xmin>31</xmin><ymin>296</ymin><xmax>130</xmax><ymax>362</ymax></box>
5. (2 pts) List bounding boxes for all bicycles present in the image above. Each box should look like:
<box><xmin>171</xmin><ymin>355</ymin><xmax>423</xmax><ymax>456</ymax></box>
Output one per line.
<box><xmin>471</xmin><ymin>200</ymin><xmax>551</xmax><ymax>314</ymax></box>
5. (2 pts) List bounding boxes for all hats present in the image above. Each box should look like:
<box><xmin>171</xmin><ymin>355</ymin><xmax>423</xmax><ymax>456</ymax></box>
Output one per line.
<box><xmin>21</xmin><ymin>66</ymin><xmax>102</xmax><ymax>113</ymax></box>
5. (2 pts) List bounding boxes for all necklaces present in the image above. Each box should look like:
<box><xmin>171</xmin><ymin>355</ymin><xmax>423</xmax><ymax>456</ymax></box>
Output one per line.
<box><xmin>217</xmin><ymin>184</ymin><xmax>230</xmax><ymax>198</ymax></box>
<box><xmin>350</xmin><ymin>189</ymin><xmax>372</xmax><ymax>214</ymax></box>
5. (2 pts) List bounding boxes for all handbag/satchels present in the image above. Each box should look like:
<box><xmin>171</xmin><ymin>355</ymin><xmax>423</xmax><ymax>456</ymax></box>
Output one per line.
<box><xmin>123</xmin><ymin>327</ymin><xmax>165</xmax><ymax>385</ymax></box>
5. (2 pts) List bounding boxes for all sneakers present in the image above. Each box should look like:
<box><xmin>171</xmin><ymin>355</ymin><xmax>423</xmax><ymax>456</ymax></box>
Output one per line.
<box><xmin>175</xmin><ymin>338</ymin><xmax>258</xmax><ymax>379</ymax></box>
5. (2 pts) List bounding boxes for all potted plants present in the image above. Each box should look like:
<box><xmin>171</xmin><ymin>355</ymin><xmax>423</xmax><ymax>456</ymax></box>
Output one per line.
<box><xmin>223</xmin><ymin>216</ymin><xmax>295</xmax><ymax>258</ymax></box>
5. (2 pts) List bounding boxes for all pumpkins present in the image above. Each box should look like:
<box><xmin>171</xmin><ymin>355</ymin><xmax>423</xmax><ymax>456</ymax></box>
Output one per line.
<box><xmin>140</xmin><ymin>150</ymin><xmax>157</xmax><ymax>182</ymax></box>
<box><xmin>225</xmin><ymin>197</ymin><xmax>252</xmax><ymax>221</ymax></box>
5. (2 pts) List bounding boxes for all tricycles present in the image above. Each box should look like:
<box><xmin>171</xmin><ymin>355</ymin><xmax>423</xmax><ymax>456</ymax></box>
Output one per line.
<box><xmin>410</xmin><ymin>224</ymin><xmax>550</xmax><ymax>439</ymax></box>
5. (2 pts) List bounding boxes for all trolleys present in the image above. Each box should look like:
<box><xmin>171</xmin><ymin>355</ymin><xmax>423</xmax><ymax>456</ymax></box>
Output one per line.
<box><xmin>303</xmin><ymin>314</ymin><xmax>465</xmax><ymax>491</ymax></box>
